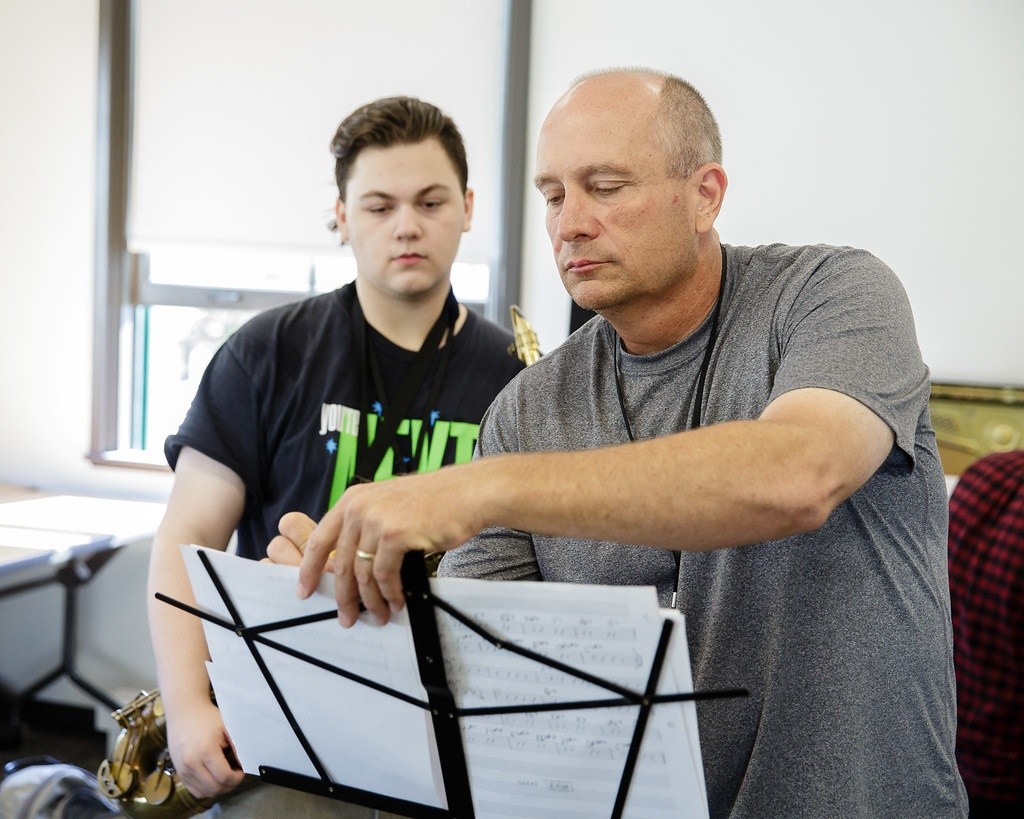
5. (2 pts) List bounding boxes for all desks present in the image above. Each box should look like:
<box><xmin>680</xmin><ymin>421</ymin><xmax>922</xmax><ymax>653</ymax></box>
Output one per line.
<box><xmin>0</xmin><ymin>483</ymin><xmax>167</xmax><ymax>761</ymax></box>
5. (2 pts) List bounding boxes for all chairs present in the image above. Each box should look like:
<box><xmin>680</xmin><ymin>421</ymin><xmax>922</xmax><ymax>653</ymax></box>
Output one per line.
<box><xmin>944</xmin><ymin>450</ymin><xmax>1024</xmax><ymax>819</ymax></box>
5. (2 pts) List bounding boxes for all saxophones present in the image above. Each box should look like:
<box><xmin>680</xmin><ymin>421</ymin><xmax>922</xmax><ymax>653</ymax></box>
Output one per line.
<box><xmin>95</xmin><ymin>303</ymin><xmax>545</xmax><ymax>819</ymax></box>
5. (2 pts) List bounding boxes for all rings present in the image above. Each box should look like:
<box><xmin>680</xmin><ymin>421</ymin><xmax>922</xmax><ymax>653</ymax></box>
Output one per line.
<box><xmin>355</xmin><ymin>550</ymin><xmax>375</xmax><ymax>561</ymax></box>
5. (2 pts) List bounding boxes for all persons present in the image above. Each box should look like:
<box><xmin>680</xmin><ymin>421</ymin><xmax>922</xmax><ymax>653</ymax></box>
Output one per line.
<box><xmin>146</xmin><ymin>96</ymin><xmax>544</xmax><ymax>819</ymax></box>
<box><xmin>258</xmin><ymin>65</ymin><xmax>969</xmax><ymax>819</ymax></box>
